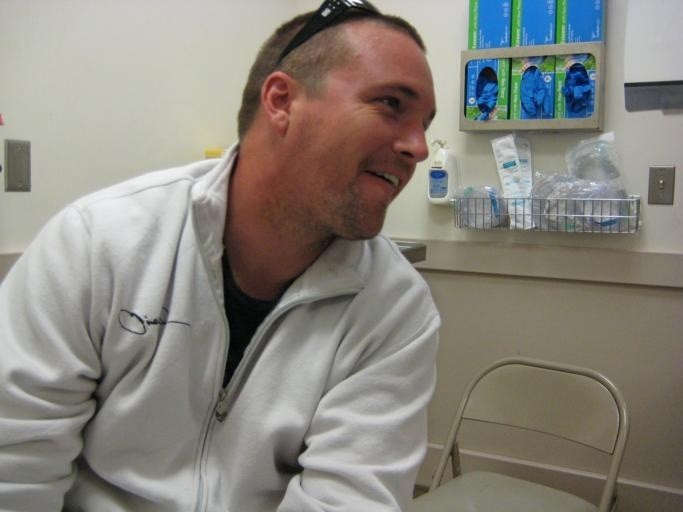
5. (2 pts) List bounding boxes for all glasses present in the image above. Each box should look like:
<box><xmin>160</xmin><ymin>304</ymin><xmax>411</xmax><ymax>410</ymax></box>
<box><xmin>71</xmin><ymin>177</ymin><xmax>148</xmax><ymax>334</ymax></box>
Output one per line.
<box><xmin>276</xmin><ymin>0</ymin><xmax>379</xmax><ymax>64</ymax></box>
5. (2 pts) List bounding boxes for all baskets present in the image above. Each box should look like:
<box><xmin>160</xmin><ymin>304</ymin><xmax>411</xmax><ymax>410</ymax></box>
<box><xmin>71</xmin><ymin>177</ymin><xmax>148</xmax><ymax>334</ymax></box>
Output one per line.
<box><xmin>453</xmin><ymin>195</ymin><xmax>640</xmax><ymax>234</ymax></box>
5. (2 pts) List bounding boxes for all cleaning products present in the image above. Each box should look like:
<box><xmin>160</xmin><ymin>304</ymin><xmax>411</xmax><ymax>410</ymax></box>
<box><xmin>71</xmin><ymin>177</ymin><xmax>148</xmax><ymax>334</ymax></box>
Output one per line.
<box><xmin>426</xmin><ymin>138</ymin><xmax>454</xmax><ymax>205</ymax></box>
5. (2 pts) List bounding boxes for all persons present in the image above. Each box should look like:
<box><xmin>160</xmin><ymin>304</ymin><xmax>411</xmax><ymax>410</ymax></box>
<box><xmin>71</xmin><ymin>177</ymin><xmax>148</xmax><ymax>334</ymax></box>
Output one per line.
<box><xmin>0</xmin><ymin>0</ymin><xmax>443</xmax><ymax>511</ymax></box>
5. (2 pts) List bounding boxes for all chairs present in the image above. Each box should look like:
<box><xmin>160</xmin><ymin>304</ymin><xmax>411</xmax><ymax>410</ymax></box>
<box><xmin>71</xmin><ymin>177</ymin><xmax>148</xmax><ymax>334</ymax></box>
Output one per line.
<box><xmin>406</xmin><ymin>355</ymin><xmax>632</xmax><ymax>512</ymax></box>
